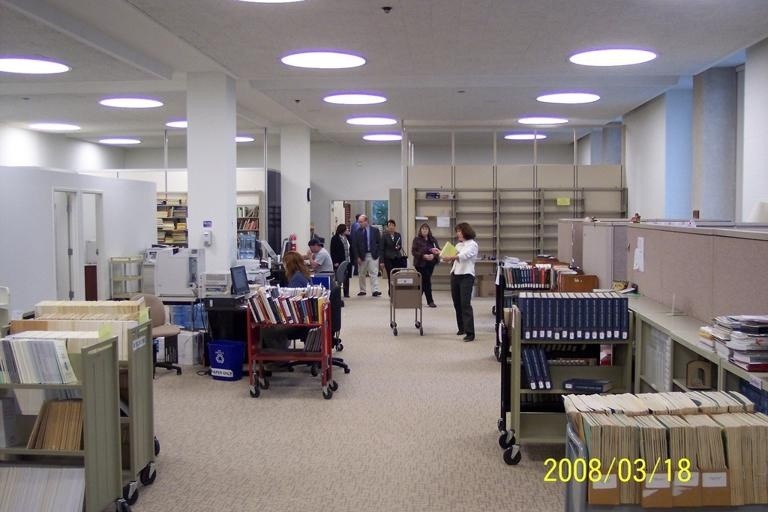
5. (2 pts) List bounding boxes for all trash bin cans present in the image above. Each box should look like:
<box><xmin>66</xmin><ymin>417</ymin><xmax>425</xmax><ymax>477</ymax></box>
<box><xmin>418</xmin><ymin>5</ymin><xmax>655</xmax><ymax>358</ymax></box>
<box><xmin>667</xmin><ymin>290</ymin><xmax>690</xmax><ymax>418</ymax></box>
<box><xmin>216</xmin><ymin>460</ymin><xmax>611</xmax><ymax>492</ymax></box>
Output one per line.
<box><xmin>207</xmin><ymin>339</ymin><xmax>246</xmax><ymax>381</ymax></box>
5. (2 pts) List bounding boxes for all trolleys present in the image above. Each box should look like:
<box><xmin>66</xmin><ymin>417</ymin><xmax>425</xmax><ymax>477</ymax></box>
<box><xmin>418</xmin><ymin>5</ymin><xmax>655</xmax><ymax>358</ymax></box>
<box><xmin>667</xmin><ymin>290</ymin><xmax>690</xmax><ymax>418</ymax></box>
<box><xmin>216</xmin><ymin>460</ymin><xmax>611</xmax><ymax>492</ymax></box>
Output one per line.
<box><xmin>560</xmin><ymin>421</ymin><xmax>768</xmax><ymax>511</ymax></box>
<box><xmin>0</xmin><ymin>285</ymin><xmax>165</xmax><ymax>511</ymax></box>
<box><xmin>242</xmin><ymin>289</ymin><xmax>339</xmax><ymax>400</ymax></box>
<box><xmin>490</xmin><ymin>261</ymin><xmax>635</xmax><ymax>468</ymax></box>
<box><xmin>389</xmin><ymin>267</ymin><xmax>424</xmax><ymax>337</ymax></box>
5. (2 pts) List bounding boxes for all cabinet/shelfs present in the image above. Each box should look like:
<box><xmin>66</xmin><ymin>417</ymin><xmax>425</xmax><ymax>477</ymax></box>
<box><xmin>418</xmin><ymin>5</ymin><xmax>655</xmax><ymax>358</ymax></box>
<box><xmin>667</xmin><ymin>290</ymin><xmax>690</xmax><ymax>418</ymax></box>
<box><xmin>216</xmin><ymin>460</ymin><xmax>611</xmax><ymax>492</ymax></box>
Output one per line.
<box><xmin>633</xmin><ymin>310</ymin><xmax>725</xmax><ymax>394</ymax></box>
<box><xmin>110</xmin><ymin>255</ymin><xmax>143</xmax><ymax>300</ymax></box>
<box><xmin>414</xmin><ymin>186</ymin><xmax>628</xmax><ymax>291</ymax></box>
<box><xmin>157</xmin><ymin>191</ymin><xmax>266</xmax><ymax>260</ymax></box>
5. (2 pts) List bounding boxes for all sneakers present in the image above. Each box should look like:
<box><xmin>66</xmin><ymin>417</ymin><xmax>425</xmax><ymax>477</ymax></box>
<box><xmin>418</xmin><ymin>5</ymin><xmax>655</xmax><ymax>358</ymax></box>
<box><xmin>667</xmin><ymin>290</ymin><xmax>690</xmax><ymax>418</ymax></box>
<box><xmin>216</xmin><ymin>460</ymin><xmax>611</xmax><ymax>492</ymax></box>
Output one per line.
<box><xmin>457</xmin><ymin>329</ymin><xmax>464</xmax><ymax>335</ymax></box>
<box><xmin>344</xmin><ymin>295</ymin><xmax>350</xmax><ymax>297</ymax></box>
<box><xmin>463</xmin><ymin>334</ymin><xmax>474</xmax><ymax>342</ymax></box>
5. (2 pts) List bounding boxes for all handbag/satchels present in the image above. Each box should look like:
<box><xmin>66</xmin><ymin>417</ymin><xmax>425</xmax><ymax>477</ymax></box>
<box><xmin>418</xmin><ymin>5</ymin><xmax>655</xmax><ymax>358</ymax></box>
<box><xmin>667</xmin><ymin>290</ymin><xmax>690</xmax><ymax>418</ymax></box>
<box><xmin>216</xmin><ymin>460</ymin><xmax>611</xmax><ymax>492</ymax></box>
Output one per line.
<box><xmin>397</xmin><ymin>246</ymin><xmax>408</xmax><ymax>268</ymax></box>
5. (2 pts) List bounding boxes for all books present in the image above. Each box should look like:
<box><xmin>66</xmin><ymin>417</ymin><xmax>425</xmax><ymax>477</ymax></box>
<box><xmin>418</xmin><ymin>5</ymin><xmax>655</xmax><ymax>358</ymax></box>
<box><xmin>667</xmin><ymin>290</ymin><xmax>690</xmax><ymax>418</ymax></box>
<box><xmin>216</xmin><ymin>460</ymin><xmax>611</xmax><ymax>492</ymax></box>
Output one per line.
<box><xmin>440</xmin><ymin>238</ymin><xmax>458</xmax><ymax>262</ymax></box>
<box><xmin>237</xmin><ymin>204</ymin><xmax>259</xmax><ymax>260</ymax></box>
<box><xmin>503</xmin><ymin>256</ymin><xmax>632</xmax><ymax>408</ymax></box>
<box><xmin>0</xmin><ymin>297</ymin><xmax>149</xmax><ymax>512</ymax></box>
<box><xmin>560</xmin><ymin>380</ymin><xmax>767</xmax><ymax>506</ymax></box>
<box><xmin>156</xmin><ymin>198</ymin><xmax>188</xmax><ymax>247</ymax></box>
<box><xmin>700</xmin><ymin>312</ymin><xmax>767</xmax><ymax>374</ymax></box>
<box><xmin>244</xmin><ymin>286</ymin><xmax>332</xmax><ymax>350</ymax></box>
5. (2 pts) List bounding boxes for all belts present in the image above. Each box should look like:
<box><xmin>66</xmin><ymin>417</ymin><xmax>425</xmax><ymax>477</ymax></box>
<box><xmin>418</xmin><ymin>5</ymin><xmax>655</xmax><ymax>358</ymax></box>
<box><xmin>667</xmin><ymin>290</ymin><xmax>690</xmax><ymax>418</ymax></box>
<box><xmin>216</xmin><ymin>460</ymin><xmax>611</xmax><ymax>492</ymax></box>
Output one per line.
<box><xmin>319</xmin><ymin>271</ymin><xmax>334</xmax><ymax>273</ymax></box>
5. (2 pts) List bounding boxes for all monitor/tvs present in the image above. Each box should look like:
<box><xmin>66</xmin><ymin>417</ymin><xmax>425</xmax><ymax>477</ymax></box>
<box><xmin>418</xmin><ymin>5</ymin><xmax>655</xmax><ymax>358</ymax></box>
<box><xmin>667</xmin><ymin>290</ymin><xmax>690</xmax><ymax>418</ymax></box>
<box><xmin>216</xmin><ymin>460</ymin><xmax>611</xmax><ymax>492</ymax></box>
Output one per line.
<box><xmin>230</xmin><ymin>265</ymin><xmax>250</xmax><ymax>295</ymax></box>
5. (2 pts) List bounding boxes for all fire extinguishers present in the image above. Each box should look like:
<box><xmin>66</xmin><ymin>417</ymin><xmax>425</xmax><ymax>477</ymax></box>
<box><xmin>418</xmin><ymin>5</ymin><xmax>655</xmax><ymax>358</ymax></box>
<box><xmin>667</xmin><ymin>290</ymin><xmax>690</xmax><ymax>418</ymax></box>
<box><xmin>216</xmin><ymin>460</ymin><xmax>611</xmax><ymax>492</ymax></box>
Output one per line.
<box><xmin>290</xmin><ymin>234</ymin><xmax>297</xmax><ymax>251</ymax></box>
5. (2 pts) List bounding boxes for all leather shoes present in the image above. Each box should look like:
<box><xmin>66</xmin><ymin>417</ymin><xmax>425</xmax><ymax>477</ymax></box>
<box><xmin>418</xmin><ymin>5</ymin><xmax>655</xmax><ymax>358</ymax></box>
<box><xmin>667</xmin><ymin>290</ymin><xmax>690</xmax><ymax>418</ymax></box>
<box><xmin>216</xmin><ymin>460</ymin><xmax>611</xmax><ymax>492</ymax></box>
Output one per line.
<box><xmin>372</xmin><ymin>291</ymin><xmax>382</xmax><ymax>296</ymax></box>
<box><xmin>429</xmin><ymin>302</ymin><xmax>436</xmax><ymax>307</ymax></box>
<box><xmin>357</xmin><ymin>292</ymin><xmax>366</xmax><ymax>296</ymax></box>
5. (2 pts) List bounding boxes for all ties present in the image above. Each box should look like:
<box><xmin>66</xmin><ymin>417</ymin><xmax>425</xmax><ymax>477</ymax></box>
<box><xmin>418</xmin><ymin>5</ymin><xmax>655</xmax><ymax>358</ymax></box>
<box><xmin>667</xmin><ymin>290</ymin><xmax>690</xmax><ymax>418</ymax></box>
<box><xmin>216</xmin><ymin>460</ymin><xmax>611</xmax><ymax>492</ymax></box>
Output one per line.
<box><xmin>363</xmin><ymin>228</ymin><xmax>368</xmax><ymax>253</ymax></box>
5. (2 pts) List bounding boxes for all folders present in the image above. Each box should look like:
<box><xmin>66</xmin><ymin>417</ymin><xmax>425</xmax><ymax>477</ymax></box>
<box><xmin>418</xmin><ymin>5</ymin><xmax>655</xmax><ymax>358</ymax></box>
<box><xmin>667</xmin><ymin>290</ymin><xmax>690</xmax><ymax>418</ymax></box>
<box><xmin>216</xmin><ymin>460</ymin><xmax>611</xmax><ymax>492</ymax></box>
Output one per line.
<box><xmin>562</xmin><ymin>378</ymin><xmax>614</xmax><ymax>393</ymax></box>
<box><xmin>517</xmin><ymin>292</ymin><xmax>628</xmax><ymax>340</ymax></box>
<box><xmin>522</xmin><ymin>348</ymin><xmax>552</xmax><ymax>390</ymax></box>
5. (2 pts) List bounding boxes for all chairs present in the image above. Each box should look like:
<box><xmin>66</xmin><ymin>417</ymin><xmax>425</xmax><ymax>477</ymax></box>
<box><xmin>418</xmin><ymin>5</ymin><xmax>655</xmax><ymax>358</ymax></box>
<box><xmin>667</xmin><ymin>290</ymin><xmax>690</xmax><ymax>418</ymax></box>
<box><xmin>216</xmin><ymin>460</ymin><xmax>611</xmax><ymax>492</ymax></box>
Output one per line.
<box><xmin>333</xmin><ymin>260</ymin><xmax>350</xmax><ymax>307</ymax></box>
<box><xmin>129</xmin><ymin>294</ymin><xmax>182</xmax><ymax>378</ymax></box>
<box><xmin>288</xmin><ymin>287</ymin><xmax>350</xmax><ymax>376</ymax></box>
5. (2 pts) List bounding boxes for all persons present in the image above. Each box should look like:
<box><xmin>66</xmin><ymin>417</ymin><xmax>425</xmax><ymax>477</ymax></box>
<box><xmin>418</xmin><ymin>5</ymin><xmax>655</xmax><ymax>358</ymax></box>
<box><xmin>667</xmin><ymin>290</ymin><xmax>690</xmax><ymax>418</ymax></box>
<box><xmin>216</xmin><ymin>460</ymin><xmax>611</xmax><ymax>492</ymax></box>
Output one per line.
<box><xmin>440</xmin><ymin>221</ymin><xmax>479</xmax><ymax>344</ymax></box>
<box><xmin>306</xmin><ymin>240</ymin><xmax>333</xmax><ymax>276</ymax></box>
<box><xmin>329</xmin><ymin>225</ymin><xmax>353</xmax><ymax>297</ymax></box>
<box><xmin>353</xmin><ymin>214</ymin><xmax>383</xmax><ymax>296</ymax></box>
<box><xmin>281</xmin><ymin>253</ymin><xmax>313</xmax><ymax>286</ymax></box>
<box><xmin>411</xmin><ymin>223</ymin><xmax>441</xmax><ymax>308</ymax></box>
<box><xmin>379</xmin><ymin>220</ymin><xmax>403</xmax><ymax>297</ymax></box>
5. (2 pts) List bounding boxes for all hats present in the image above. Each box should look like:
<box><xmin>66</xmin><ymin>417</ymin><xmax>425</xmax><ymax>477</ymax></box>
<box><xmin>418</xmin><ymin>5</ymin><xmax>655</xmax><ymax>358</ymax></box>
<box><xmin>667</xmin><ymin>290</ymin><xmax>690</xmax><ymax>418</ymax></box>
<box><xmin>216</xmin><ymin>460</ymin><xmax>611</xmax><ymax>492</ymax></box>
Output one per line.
<box><xmin>308</xmin><ymin>239</ymin><xmax>324</xmax><ymax>247</ymax></box>
